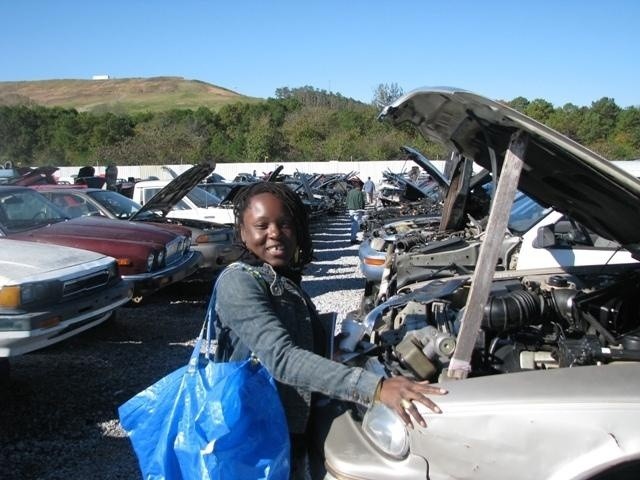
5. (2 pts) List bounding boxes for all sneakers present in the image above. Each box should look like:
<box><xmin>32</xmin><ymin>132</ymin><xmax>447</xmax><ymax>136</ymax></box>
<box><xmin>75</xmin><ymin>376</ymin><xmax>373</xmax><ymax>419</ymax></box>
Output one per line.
<box><xmin>351</xmin><ymin>240</ymin><xmax>361</xmax><ymax>244</ymax></box>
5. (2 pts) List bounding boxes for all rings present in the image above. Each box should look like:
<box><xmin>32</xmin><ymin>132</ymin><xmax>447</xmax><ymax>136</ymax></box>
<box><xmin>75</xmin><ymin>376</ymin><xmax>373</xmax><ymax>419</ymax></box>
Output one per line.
<box><xmin>402</xmin><ymin>400</ymin><xmax>411</xmax><ymax>409</ymax></box>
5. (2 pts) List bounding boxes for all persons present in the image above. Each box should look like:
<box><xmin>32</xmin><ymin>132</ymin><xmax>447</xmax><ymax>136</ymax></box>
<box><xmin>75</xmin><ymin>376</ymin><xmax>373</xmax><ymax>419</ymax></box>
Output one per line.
<box><xmin>346</xmin><ymin>181</ymin><xmax>364</xmax><ymax>244</ymax></box>
<box><xmin>213</xmin><ymin>182</ymin><xmax>448</xmax><ymax>480</ymax></box>
<box><xmin>364</xmin><ymin>177</ymin><xmax>375</xmax><ymax>204</ymax></box>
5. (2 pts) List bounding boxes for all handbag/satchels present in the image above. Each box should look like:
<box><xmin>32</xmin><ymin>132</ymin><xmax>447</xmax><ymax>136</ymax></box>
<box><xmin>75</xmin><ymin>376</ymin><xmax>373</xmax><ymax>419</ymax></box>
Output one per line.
<box><xmin>118</xmin><ymin>261</ymin><xmax>291</xmax><ymax>480</ymax></box>
